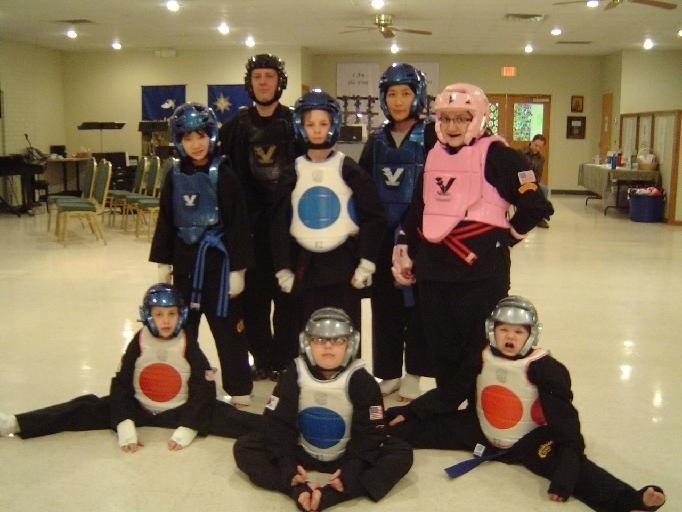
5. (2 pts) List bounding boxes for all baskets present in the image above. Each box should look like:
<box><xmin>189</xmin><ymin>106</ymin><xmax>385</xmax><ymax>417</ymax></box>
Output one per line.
<box><xmin>636</xmin><ymin>147</ymin><xmax>658</xmax><ymax>170</ymax></box>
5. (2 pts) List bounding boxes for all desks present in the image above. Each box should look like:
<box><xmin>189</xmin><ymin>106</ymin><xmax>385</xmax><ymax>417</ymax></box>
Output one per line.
<box><xmin>572</xmin><ymin>160</ymin><xmax>661</xmax><ymax>219</ymax></box>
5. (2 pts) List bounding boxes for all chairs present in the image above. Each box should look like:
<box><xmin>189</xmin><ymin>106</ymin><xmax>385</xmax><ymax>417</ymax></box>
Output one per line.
<box><xmin>38</xmin><ymin>150</ymin><xmax>183</xmax><ymax>249</ymax></box>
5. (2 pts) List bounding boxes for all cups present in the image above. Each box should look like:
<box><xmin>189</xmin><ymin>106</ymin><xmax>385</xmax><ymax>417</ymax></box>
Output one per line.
<box><xmin>48</xmin><ymin>152</ymin><xmax>90</xmax><ymax>160</ymax></box>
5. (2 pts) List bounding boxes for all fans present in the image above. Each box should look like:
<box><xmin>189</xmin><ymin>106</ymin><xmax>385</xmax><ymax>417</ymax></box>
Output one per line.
<box><xmin>551</xmin><ymin>1</ymin><xmax>679</xmax><ymax>14</ymax></box>
<box><xmin>336</xmin><ymin>13</ymin><xmax>433</xmax><ymax>41</ymax></box>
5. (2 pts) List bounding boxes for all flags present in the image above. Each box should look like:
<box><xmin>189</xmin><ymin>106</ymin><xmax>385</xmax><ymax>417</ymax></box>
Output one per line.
<box><xmin>142</xmin><ymin>86</ymin><xmax>184</xmax><ymax>121</ymax></box>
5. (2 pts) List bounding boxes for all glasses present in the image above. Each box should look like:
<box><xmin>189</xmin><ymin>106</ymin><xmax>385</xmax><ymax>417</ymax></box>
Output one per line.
<box><xmin>438</xmin><ymin>116</ymin><xmax>472</xmax><ymax>125</ymax></box>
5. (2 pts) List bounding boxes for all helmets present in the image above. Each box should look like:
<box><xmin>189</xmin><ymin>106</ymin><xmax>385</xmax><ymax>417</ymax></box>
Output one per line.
<box><xmin>170</xmin><ymin>102</ymin><xmax>220</xmax><ymax>158</ymax></box>
<box><xmin>298</xmin><ymin>307</ymin><xmax>361</xmax><ymax>369</ymax></box>
<box><xmin>293</xmin><ymin>89</ymin><xmax>340</xmax><ymax>148</ymax></box>
<box><xmin>484</xmin><ymin>296</ymin><xmax>540</xmax><ymax>357</ymax></box>
<box><xmin>136</xmin><ymin>283</ymin><xmax>189</xmax><ymax>338</ymax></box>
<box><xmin>245</xmin><ymin>54</ymin><xmax>287</xmax><ymax>105</ymax></box>
<box><xmin>378</xmin><ymin>63</ymin><xmax>427</xmax><ymax>122</ymax></box>
<box><xmin>430</xmin><ymin>83</ymin><xmax>491</xmax><ymax>147</ymax></box>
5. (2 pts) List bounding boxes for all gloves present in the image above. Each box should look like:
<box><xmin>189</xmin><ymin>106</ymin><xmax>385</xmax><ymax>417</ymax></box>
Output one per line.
<box><xmin>351</xmin><ymin>257</ymin><xmax>376</xmax><ymax>290</ymax></box>
<box><xmin>390</xmin><ymin>244</ymin><xmax>417</xmax><ymax>287</ymax></box>
<box><xmin>275</xmin><ymin>268</ymin><xmax>296</xmax><ymax>294</ymax></box>
<box><xmin>228</xmin><ymin>267</ymin><xmax>247</xmax><ymax>299</ymax></box>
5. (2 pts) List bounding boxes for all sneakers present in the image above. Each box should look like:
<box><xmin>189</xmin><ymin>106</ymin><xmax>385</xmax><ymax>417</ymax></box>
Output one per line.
<box><xmin>268</xmin><ymin>361</ymin><xmax>289</xmax><ymax>383</ymax></box>
<box><xmin>250</xmin><ymin>364</ymin><xmax>271</xmax><ymax>380</ymax></box>
<box><xmin>536</xmin><ymin>219</ymin><xmax>549</xmax><ymax>228</ymax></box>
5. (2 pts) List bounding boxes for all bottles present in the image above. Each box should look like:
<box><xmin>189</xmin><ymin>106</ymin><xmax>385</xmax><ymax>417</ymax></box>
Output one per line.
<box><xmin>592</xmin><ymin>149</ymin><xmax>657</xmax><ymax>169</ymax></box>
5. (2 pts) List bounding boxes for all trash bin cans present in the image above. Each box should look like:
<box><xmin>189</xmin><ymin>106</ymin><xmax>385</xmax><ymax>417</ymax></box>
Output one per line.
<box><xmin>627</xmin><ymin>192</ymin><xmax>666</xmax><ymax>222</ymax></box>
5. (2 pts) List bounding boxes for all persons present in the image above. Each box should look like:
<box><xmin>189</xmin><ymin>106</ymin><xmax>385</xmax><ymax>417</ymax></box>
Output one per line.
<box><xmin>0</xmin><ymin>284</ymin><xmax>263</xmax><ymax>452</ymax></box>
<box><xmin>268</xmin><ymin>89</ymin><xmax>385</xmax><ymax>369</ymax></box>
<box><xmin>391</xmin><ymin>83</ymin><xmax>554</xmax><ymax>412</ymax></box>
<box><xmin>233</xmin><ymin>306</ymin><xmax>413</xmax><ymax>512</ymax></box>
<box><xmin>386</xmin><ymin>297</ymin><xmax>666</xmax><ymax>512</ymax></box>
<box><xmin>216</xmin><ymin>55</ymin><xmax>306</xmax><ymax>382</ymax></box>
<box><xmin>358</xmin><ymin>64</ymin><xmax>438</xmax><ymax>401</ymax></box>
<box><xmin>516</xmin><ymin>135</ymin><xmax>550</xmax><ymax>228</ymax></box>
<box><xmin>148</xmin><ymin>103</ymin><xmax>254</xmax><ymax>406</ymax></box>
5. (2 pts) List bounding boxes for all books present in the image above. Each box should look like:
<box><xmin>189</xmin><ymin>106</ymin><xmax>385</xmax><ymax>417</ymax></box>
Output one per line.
<box><xmin>208</xmin><ymin>85</ymin><xmax>253</xmax><ymax>122</ymax></box>
